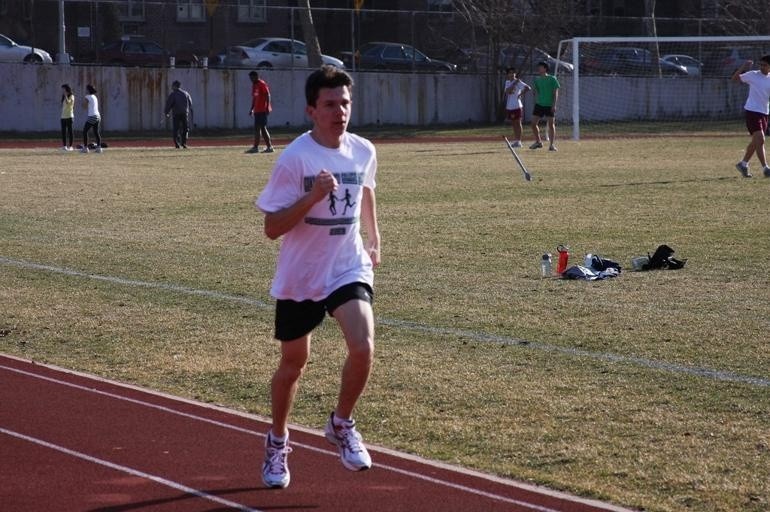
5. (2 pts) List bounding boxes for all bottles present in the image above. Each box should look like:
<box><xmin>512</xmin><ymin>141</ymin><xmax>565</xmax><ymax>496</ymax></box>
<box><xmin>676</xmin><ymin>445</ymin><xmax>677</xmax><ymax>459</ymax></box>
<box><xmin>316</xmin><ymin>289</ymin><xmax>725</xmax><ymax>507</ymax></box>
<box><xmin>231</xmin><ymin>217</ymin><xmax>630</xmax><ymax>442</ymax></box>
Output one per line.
<box><xmin>541</xmin><ymin>254</ymin><xmax>552</xmax><ymax>278</ymax></box>
<box><xmin>557</xmin><ymin>244</ymin><xmax>570</xmax><ymax>274</ymax></box>
<box><xmin>585</xmin><ymin>254</ymin><xmax>593</xmax><ymax>267</ymax></box>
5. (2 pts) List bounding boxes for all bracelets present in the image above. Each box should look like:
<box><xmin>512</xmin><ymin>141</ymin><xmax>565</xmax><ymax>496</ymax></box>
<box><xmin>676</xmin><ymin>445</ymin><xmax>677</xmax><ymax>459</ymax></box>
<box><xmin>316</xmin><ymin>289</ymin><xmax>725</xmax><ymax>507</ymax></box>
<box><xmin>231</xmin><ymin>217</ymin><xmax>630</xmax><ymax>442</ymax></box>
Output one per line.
<box><xmin>248</xmin><ymin>109</ymin><xmax>253</xmax><ymax>112</ymax></box>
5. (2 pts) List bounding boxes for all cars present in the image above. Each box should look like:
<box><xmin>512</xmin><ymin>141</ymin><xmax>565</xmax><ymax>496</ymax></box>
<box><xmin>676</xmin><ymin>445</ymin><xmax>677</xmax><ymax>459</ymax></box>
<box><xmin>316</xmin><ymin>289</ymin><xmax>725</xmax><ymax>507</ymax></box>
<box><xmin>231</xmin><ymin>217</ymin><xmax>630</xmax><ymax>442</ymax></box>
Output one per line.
<box><xmin>340</xmin><ymin>42</ymin><xmax>456</xmax><ymax>72</ymax></box>
<box><xmin>0</xmin><ymin>33</ymin><xmax>54</xmax><ymax>64</ymax></box>
<box><xmin>469</xmin><ymin>41</ymin><xmax>765</xmax><ymax>76</ymax></box>
<box><xmin>223</xmin><ymin>37</ymin><xmax>346</xmax><ymax>70</ymax></box>
<box><xmin>88</xmin><ymin>34</ymin><xmax>200</xmax><ymax>67</ymax></box>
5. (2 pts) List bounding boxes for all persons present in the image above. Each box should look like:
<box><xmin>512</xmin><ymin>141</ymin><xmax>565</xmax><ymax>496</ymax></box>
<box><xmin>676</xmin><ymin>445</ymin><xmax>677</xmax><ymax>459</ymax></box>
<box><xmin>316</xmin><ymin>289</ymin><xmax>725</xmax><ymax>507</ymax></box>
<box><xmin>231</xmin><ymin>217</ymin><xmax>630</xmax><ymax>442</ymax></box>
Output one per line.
<box><xmin>730</xmin><ymin>55</ymin><xmax>770</xmax><ymax>179</ymax></box>
<box><xmin>504</xmin><ymin>66</ymin><xmax>530</xmax><ymax>149</ymax></box>
<box><xmin>529</xmin><ymin>62</ymin><xmax>561</xmax><ymax>151</ymax></box>
<box><xmin>243</xmin><ymin>70</ymin><xmax>275</xmax><ymax>154</ymax></box>
<box><xmin>59</xmin><ymin>83</ymin><xmax>75</xmax><ymax>151</ymax></box>
<box><xmin>80</xmin><ymin>84</ymin><xmax>102</xmax><ymax>152</ymax></box>
<box><xmin>255</xmin><ymin>66</ymin><xmax>383</xmax><ymax>490</ymax></box>
<box><xmin>164</xmin><ymin>79</ymin><xmax>195</xmax><ymax>147</ymax></box>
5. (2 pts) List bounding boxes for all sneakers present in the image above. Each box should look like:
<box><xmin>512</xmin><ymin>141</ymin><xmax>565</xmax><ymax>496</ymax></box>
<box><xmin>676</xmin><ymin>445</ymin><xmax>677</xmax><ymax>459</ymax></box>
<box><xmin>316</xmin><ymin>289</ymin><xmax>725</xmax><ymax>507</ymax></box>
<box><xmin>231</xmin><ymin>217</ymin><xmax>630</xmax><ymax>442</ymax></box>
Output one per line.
<box><xmin>244</xmin><ymin>146</ymin><xmax>259</xmax><ymax>153</ymax></box>
<box><xmin>62</xmin><ymin>145</ymin><xmax>103</xmax><ymax>154</ymax></box>
<box><xmin>736</xmin><ymin>161</ymin><xmax>753</xmax><ymax>178</ymax></box>
<box><xmin>763</xmin><ymin>167</ymin><xmax>770</xmax><ymax>178</ymax></box>
<box><xmin>260</xmin><ymin>426</ymin><xmax>294</xmax><ymax>490</ymax></box>
<box><xmin>263</xmin><ymin>147</ymin><xmax>275</xmax><ymax>152</ymax></box>
<box><xmin>510</xmin><ymin>140</ymin><xmax>522</xmax><ymax>148</ymax></box>
<box><xmin>548</xmin><ymin>144</ymin><xmax>558</xmax><ymax>152</ymax></box>
<box><xmin>323</xmin><ymin>412</ymin><xmax>373</xmax><ymax>472</ymax></box>
<box><xmin>174</xmin><ymin>143</ymin><xmax>187</xmax><ymax>149</ymax></box>
<box><xmin>529</xmin><ymin>141</ymin><xmax>543</xmax><ymax>149</ymax></box>
